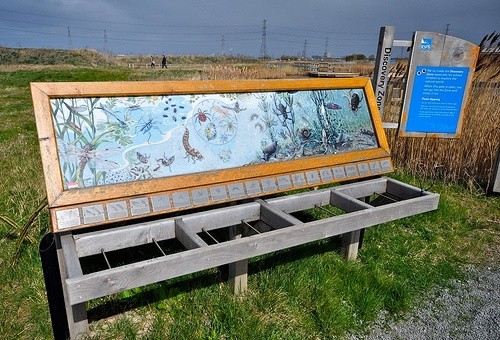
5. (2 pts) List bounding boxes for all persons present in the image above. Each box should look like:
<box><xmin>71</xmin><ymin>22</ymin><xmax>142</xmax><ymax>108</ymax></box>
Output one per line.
<box><xmin>161</xmin><ymin>54</ymin><xmax>168</xmax><ymax>69</ymax></box>
<box><xmin>151</xmin><ymin>62</ymin><xmax>155</xmax><ymax>68</ymax></box>
